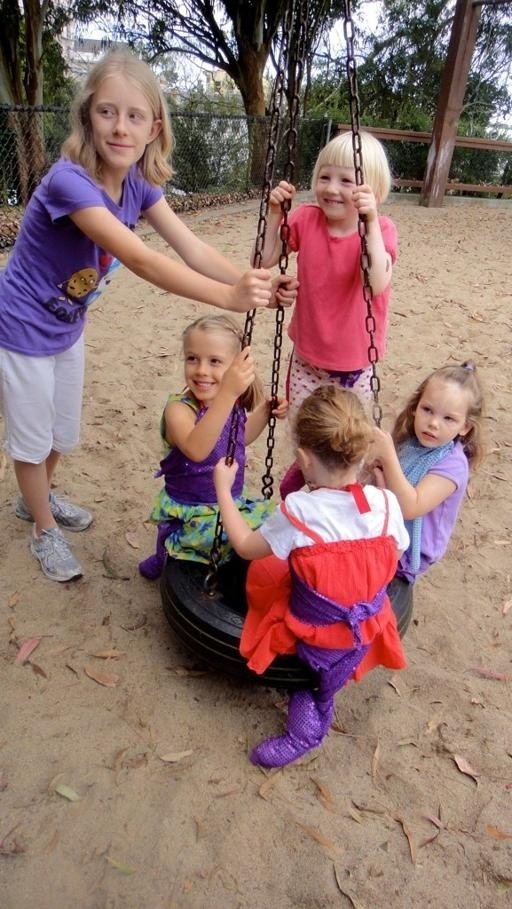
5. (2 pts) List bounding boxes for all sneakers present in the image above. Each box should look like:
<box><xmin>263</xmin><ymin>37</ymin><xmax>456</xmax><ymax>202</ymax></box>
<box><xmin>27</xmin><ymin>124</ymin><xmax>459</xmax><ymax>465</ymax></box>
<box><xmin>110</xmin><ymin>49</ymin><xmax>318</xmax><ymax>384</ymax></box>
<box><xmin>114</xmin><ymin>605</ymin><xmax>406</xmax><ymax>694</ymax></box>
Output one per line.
<box><xmin>14</xmin><ymin>490</ymin><xmax>93</xmax><ymax>533</ymax></box>
<box><xmin>30</xmin><ymin>522</ymin><xmax>83</xmax><ymax>583</ymax></box>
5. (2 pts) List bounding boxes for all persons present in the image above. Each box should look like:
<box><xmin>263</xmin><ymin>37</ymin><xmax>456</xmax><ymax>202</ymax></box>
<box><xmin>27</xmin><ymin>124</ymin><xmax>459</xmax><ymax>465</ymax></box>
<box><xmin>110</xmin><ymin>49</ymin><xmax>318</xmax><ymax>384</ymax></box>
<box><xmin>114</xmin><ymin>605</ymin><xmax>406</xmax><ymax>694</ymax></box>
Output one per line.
<box><xmin>356</xmin><ymin>357</ymin><xmax>483</xmax><ymax>586</ymax></box>
<box><xmin>1</xmin><ymin>50</ymin><xmax>301</xmax><ymax>585</ymax></box>
<box><xmin>209</xmin><ymin>386</ymin><xmax>412</xmax><ymax>698</ymax></box>
<box><xmin>142</xmin><ymin>311</ymin><xmax>290</xmax><ymax>576</ymax></box>
<box><xmin>249</xmin><ymin>125</ymin><xmax>401</xmax><ymax>499</ymax></box>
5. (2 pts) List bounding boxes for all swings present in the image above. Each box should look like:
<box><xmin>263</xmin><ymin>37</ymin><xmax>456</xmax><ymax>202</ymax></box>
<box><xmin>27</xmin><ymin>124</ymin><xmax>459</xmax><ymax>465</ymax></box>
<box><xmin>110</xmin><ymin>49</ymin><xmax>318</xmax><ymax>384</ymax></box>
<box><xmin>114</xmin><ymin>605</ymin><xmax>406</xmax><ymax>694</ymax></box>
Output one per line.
<box><xmin>166</xmin><ymin>0</ymin><xmax>411</xmax><ymax>681</ymax></box>
